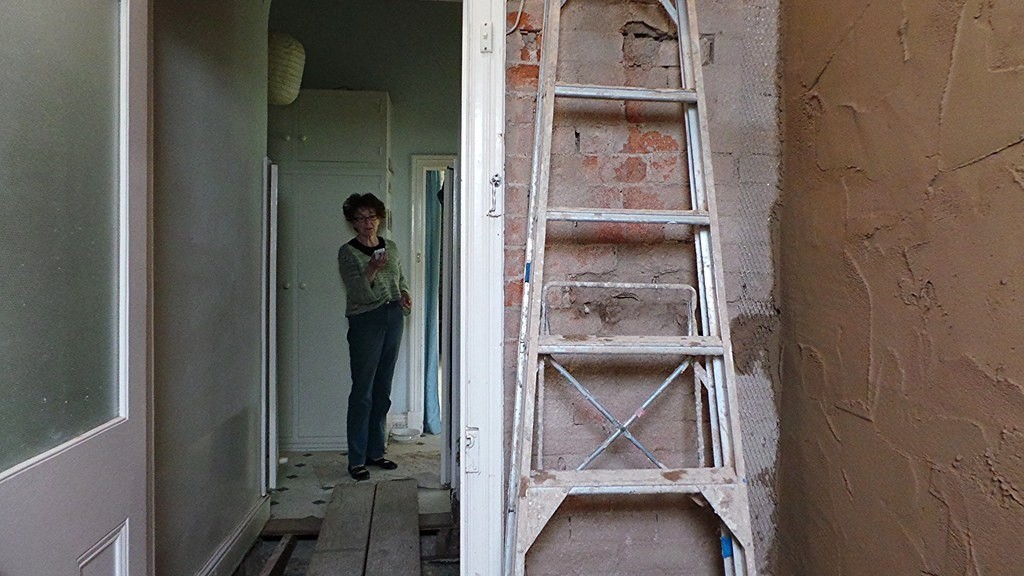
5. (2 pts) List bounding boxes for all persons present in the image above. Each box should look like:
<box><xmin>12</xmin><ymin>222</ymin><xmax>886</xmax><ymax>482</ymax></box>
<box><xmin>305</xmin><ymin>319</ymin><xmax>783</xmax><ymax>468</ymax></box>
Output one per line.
<box><xmin>338</xmin><ymin>192</ymin><xmax>412</xmax><ymax>481</ymax></box>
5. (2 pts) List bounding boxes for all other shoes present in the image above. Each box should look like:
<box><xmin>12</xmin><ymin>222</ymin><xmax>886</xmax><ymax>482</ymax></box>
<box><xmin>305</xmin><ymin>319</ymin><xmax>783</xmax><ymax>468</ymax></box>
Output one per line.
<box><xmin>365</xmin><ymin>456</ymin><xmax>397</xmax><ymax>469</ymax></box>
<box><xmin>348</xmin><ymin>464</ymin><xmax>370</xmax><ymax>480</ymax></box>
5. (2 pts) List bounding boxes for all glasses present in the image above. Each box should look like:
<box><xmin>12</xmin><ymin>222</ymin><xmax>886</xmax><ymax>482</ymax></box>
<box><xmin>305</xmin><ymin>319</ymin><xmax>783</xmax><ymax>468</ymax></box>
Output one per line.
<box><xmin>353</xmin><ymin>214</ymin><xmax>378</xmax><ymax>223</ymax></box>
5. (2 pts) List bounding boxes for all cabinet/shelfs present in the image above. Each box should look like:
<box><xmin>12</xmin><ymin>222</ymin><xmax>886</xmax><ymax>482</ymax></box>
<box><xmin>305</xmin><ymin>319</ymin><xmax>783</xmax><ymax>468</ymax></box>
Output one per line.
<box><xmin>276</xmin><ymin>171</ymin><xmax>394</xmax><ymax>452</ymax></box>
<box><xmin>266</xmin><ymin>88</ymin><xmax>395</xmax><ymax>175</ymax></box>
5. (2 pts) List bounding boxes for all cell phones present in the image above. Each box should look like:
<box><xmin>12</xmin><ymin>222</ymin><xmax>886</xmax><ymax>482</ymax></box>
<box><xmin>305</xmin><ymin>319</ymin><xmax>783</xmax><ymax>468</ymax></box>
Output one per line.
<box><xmin>375</xmin><ymin>248</ymin><xmax>386</xmax><ymax>260</ymax></box>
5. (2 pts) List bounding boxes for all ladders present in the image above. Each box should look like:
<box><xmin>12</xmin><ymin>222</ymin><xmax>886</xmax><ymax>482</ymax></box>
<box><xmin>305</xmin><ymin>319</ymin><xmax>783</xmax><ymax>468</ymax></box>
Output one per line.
<box><xmin>503</xmin><ymin>1</ymin><xmax>757</xmax><ymax>576</ymax></box>
